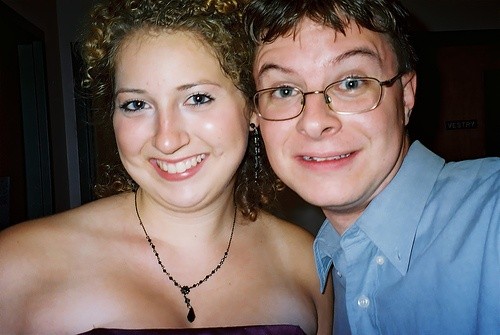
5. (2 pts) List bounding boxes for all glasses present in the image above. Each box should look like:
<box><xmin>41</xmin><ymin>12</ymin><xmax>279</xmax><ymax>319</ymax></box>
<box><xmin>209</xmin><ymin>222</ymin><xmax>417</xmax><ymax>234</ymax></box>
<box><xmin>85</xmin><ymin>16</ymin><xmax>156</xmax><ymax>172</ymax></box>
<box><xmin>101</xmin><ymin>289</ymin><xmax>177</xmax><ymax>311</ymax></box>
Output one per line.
<box><xmin>253</xmin><ymin>67</ymin><xmax>409</xmax><ymax>121</ymax></box>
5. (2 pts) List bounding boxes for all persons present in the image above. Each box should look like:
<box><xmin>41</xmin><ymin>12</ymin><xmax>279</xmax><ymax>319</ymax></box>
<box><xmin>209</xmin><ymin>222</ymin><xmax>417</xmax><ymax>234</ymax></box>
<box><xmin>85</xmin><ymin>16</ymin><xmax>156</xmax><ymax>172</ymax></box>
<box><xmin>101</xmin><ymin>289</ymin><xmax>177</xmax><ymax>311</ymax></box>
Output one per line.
<box><xmin>0</xmin><ymin>1</ymin><xmax>335</xmax><ymax>335</ymax></box>
<box><xmin>240</xmin><ymin>0</ymin><xmax>500</xmax><ymax>335</ymax></box>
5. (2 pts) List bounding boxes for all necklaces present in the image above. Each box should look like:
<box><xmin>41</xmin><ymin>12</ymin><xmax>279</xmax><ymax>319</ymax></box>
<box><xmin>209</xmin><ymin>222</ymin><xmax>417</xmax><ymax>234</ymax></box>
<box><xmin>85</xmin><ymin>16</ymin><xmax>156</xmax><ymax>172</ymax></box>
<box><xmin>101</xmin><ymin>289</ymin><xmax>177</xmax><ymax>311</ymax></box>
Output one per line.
<box><xmin>134</xmin><ymin>186</ymin><xmax>237</xmax><ymax>323</ymax></box>
